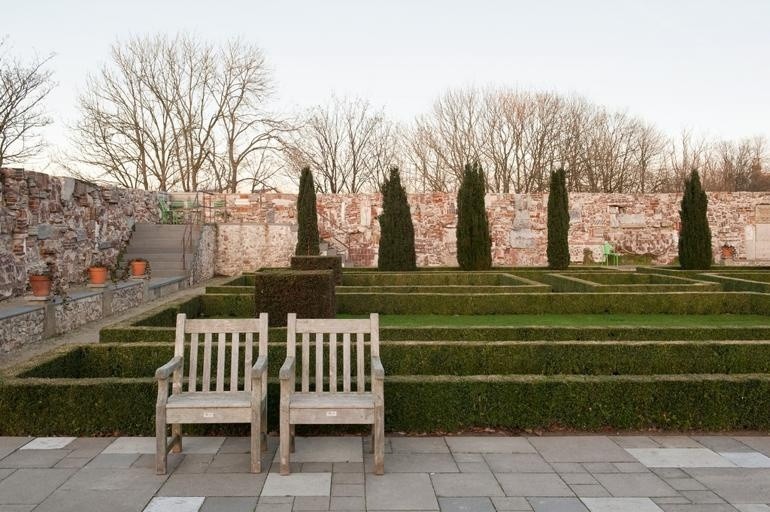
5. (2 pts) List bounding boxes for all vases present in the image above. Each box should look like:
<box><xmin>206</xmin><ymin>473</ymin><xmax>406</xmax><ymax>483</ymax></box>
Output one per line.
<box><xmin>30</xmin><ymin>260</ymin><xmax>146</xmax><ymax>296</ymax></box>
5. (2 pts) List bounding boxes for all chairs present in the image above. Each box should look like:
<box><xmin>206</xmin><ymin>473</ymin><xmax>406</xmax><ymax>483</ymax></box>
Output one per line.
<box><xmin>159</xmin><ymin>200</ymin><xmax>225</xmax><ymax>224</ymax></box>
<box><xmin>604</xmin><ymin>241</ymin><xmax>620</xmax><ymax>267</ymax></box>
<box><xmin>154</xmin><ymin>312</ymin><xmax>386</xmax><ymax>475</ymax></box>
